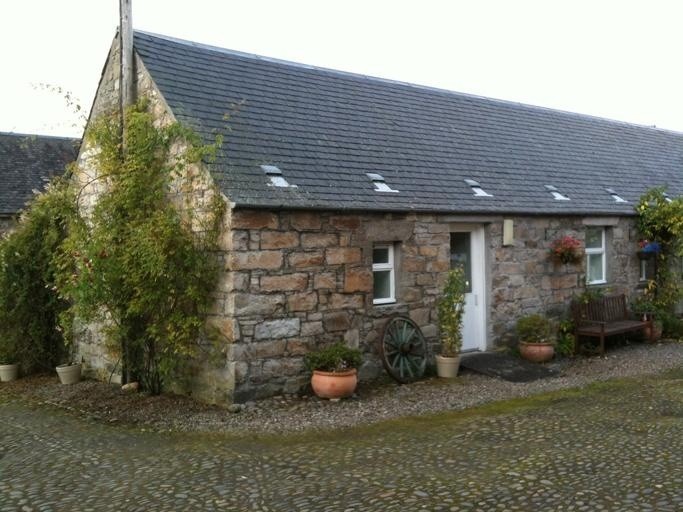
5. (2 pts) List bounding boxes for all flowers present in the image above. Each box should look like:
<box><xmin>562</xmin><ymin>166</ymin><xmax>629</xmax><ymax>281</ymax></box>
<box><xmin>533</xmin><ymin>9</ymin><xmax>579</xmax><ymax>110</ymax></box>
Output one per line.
<box><xmin>636</xmin><ymin>240</ymin><xmax>659</xmax><ymax>253</ymax></box>
<box><xmin>550</xmin><ymin>236</ymin><xmax>582</xmax><ymax>257</ymax></box>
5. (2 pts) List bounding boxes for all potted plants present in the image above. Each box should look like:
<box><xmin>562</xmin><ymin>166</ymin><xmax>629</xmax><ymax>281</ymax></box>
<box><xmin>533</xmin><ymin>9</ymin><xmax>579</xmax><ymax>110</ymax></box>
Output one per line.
<box><xmin>307</xmin><ymin>340</ymin><xmax>363</xmax><ymax>399</ymax></box>
<box><xmin>631</xmin><ymin>301</ymin><xmax>656</xmax><ymax>338</ymax></box>
<box><xmin>516</xmin><ymin>314</ymin><xmax>555</xmax><ymax>361</ymax></box>
<box><xmin>435</xmin><ymin>264</ymin><xmax>466</xmax><ymax>377</ymax></box>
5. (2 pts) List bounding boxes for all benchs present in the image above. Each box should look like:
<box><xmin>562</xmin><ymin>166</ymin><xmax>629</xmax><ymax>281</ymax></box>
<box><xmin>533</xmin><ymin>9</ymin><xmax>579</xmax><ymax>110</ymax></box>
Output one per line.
<box><xmin>571</xmin><ymin>293</ymin><xmax>654</xmax><ymax>359</ymax></box>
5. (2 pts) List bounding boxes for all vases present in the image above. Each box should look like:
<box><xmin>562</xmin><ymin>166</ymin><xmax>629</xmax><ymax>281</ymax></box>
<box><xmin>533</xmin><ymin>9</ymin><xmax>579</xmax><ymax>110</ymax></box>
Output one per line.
<box><xmin>0</xmin><ymin>364</ymin><xmax>18</xmax><ymax>382</ymax></box>
<box><xmin>56</xmin><ymin>297</ymin><xmax>87</xmax><ymax>384</ymax></box>
<box><xmin>638</xmin><ymin>253</ymin><xmax>651</xmax><ymax>259</ymax></box>
<box><xmin>651</xmin><ymin>320</ymin><xmax>663</xmax><ymax>340</ymax></box>
<box><xmin>571</xmin><ymin>248</ymin><xmax>582</xmax><ymax>257</ymax></box>
